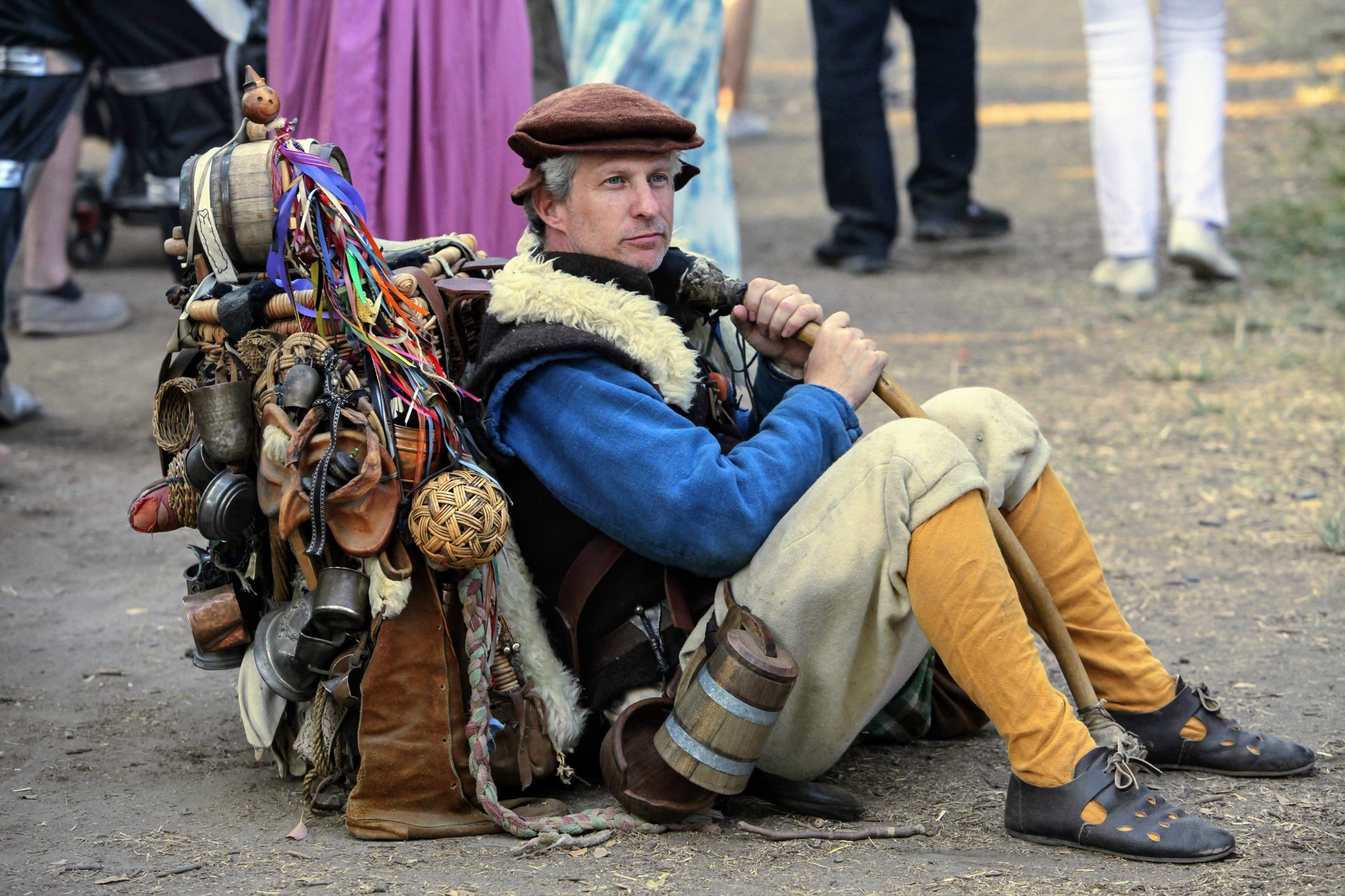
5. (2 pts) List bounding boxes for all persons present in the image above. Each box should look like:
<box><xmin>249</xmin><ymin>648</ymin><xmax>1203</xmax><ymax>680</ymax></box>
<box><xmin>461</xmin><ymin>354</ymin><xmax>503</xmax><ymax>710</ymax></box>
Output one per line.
<box><xmin>0</xmin><ymin>0</ymin><xmax>131</xmax><ymax>430</ymax></box>
<box><xmin>266</xmin><ymin>0</ymin><xmax>1240</xmax><ymax>386</ymax></box>
<box><xmin>467</xmin><ymin>84</ymin><xmax>1316</xmax><ymax>865</ymax></box>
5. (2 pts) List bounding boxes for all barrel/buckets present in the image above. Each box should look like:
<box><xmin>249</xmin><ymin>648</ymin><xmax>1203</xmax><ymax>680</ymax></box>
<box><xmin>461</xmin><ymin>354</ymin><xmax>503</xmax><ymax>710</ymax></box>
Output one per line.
<box><xmin>385</xmin><ymin>420</ymin><xmax>443</xmax><ymax>500</ymax></box>
<box><xmin>178</xmin><ymin>137</ymin><xmax>352</xmax><ymax>271</ymax></box>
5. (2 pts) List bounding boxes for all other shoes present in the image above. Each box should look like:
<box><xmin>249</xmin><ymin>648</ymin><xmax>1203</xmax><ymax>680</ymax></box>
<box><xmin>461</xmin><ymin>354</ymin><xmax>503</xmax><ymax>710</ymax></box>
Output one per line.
<box><xmin>0</xmin><ymin>371</ymin><xmax>37</xmax><ymax>424</ymax></box>
<box><xmin>1094</xmin><ymin>256</ymin><xmax>1159</xmax><ymax>298</ymax></box>
<box><xmin>1166</xmin><ymin>216</ymin><xmax>1240</xmax><ymax>279</ymax></box>
<box><xmin>19</xmin><ymin>287</ymin><xmax>131</xmax><ymax>334</ymax></box>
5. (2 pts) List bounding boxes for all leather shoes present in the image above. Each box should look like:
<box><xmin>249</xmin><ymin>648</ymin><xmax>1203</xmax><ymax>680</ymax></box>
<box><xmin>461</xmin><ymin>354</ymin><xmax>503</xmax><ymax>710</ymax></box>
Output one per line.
<box><xmin>914</xmin><ymin>197</ymin><xmax>1010</xmax><ymax>239</ymax></box>
<box><xmin>1124</xmin><ymin>696</ymin><xmax>1315</xmax><ymax>776</ymax></box>
<box><xmin>816</xmin><ymin>214</ymin><xmax>891</xmax><ymax>274</ymax></box>
<box><xmin>1004</xmin><ymin>733</ymin><xmax>1236</xmax><ymax>863</ymax></box>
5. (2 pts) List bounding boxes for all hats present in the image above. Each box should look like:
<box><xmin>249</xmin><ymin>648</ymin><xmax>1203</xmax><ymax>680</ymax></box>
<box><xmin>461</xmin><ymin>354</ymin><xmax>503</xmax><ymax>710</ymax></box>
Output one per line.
<box><xmin>507</xmin><ymin>83</ymin><xmax>705</xmax><ymax>205</ymax></box>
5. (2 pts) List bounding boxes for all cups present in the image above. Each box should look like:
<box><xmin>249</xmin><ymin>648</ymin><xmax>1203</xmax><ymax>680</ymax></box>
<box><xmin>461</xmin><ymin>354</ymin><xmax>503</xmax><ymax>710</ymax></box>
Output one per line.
<box><xmin>652</xmin><ymin>629</ymin><xmax>802</xmax><ymax>796</ymax></box>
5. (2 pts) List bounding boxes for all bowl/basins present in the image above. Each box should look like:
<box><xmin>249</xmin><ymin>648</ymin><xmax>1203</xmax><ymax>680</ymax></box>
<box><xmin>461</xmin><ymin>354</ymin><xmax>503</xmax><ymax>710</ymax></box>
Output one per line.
<box><xmin>183</xmin><ymin>441</ymin><xmax>224</xmax><ymax>495</ymax></box>
<box><xmin>197</xmin><ymin>467</ymin><xmax>257</xmax><ymax>541</ymax></box>
<box><xmin>182</xmin><ymin>583</ymin><xmax>252</xmax><ymax>653</ymax></box>
<box><xmin>312</xmin><ymin>565</ymin><xmax>370</xmax><ymax>627</ymax></box>
<box><xmin>191</xmin><ymin>650</ymin><xmax>244</xmax><ymax>671</ymax></box>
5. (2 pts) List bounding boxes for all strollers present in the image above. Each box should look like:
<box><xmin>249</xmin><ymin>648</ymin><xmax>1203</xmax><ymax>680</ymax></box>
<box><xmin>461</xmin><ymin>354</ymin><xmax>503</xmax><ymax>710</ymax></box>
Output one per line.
<box><xmin>67</xmin><ymin>0</ymin><xmax>268</xmax><ymax>273</ymax></box>
